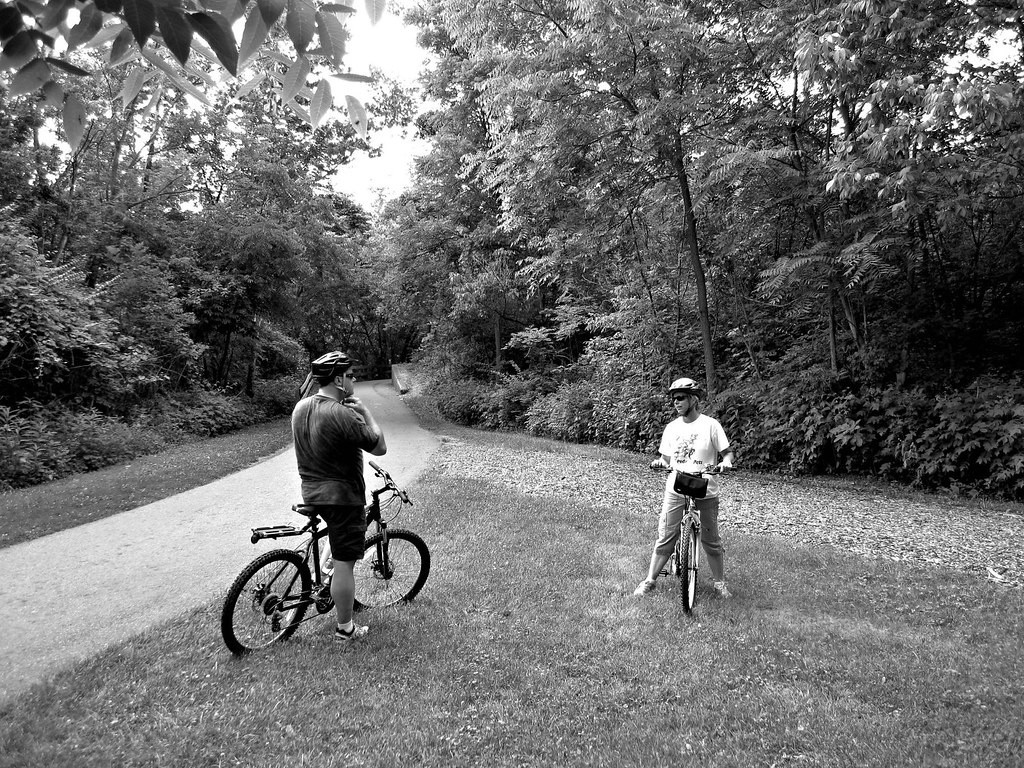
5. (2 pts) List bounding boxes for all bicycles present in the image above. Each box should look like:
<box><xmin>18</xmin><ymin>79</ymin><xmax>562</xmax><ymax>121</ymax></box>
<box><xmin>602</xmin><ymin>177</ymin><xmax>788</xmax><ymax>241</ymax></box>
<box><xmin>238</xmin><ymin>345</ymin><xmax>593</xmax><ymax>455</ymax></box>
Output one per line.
<box><xmin>221</xmin><ymin>461</ymin><xmax>430</xmax><ymax>655</ymax></box>
<box><xmin>650</xmin><ymin>464</ymin><xmax>737</xmax><ymax>613</ymax></box>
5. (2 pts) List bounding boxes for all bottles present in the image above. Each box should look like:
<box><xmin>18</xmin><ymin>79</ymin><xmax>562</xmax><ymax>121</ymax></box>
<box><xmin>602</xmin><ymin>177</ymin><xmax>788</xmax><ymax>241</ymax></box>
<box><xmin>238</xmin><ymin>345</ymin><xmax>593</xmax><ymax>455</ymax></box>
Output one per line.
<box><xmin>321</xmin><ymin>553</ymin><xmax>334</xmax><ymax>575</ymax></box>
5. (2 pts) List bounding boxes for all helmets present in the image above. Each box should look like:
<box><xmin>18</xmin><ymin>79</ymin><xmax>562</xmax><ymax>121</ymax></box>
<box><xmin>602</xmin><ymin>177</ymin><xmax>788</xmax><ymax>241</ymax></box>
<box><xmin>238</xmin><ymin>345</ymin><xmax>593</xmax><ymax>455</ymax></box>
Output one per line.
<box><xmin>668</xmin><ymin>377</ymin><xmax>703</xmax><ymax>393</ymax></box>
<box><xmin>310</xmin><ymin>351</ymin><xmax>359</xmax><ymax>378</ymax></box>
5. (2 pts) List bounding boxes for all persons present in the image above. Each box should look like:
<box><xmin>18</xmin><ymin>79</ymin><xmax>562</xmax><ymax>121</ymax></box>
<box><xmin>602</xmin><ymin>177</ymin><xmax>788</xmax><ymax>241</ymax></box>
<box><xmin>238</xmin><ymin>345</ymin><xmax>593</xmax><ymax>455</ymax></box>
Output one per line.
<box><xmin>291</xmin><ymin>351</ymin><xmax>387</xmax><ymax>643</ymax></box>
<box><xmin>634</xmin><ymin>377</ymin><xmax>735</xmax><ymax>598</ymax></box>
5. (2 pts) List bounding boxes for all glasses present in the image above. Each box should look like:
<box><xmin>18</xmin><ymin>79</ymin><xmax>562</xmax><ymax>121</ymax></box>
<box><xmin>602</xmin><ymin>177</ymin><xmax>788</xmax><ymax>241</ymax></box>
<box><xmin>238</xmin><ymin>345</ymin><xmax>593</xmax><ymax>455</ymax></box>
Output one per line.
<box><xmin>672</xmin><ymin>395</ymin><xmax>689</xmax><ymax>402</ymax></box>
<box><xmin>336</xmin><ymin>373</ymin><xmax>354</xmax><ymax>379</ymax></box>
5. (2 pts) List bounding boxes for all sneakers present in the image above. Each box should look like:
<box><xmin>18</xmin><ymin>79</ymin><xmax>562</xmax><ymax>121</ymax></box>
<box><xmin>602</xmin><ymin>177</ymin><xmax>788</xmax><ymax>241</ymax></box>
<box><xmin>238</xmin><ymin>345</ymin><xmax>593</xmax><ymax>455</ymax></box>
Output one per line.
<box><xmin>333</xmin><ymin>622</ymin><xmax>370</xmax><ymax>644</ymax></box>
<box><xmin>321</xmin><ymin>558</ymin><xmax>333</xmax><ymax>575</ymax></box>
<box><xmin>633</xmin><ymin>580</ymin><xmax>655</xmax><ymax>595</ymax></box>
<box><xmin>714</xmin><ymin>579</ymin><xmax>733</xmax><ymax>599</ymax></box>
